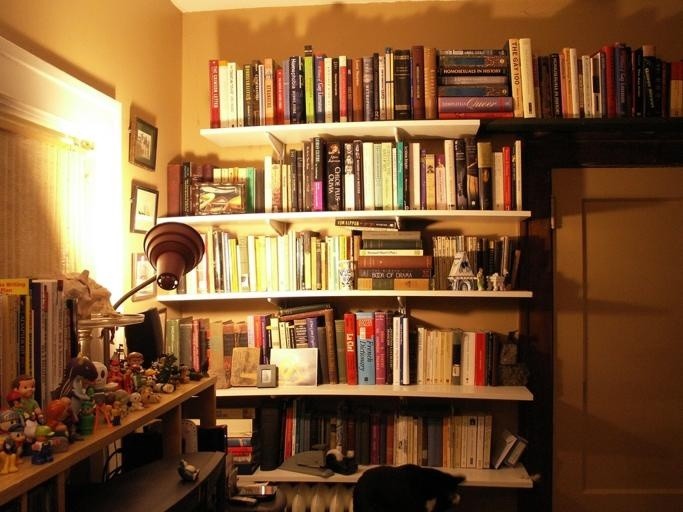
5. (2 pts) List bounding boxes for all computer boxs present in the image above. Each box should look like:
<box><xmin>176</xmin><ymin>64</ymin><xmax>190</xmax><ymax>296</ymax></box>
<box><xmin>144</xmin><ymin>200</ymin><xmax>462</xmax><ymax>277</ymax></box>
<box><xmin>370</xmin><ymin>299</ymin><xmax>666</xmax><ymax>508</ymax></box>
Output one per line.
<box><xmin>122</xmin><ymin>424</ymin><xmax>227</xmax><ymax>475</ymax></box>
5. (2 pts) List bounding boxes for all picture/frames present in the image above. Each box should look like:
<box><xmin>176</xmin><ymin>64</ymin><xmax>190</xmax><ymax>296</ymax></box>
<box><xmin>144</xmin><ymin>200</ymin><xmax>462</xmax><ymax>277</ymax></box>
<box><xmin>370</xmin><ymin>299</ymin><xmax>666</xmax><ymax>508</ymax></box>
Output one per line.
<box><xmin>193</xmin><ymin>181</ymin><xmax>248</xmax><ymax>216</ymax></box>
<box><xmin>257</xmin><ymin>364</ymin><xmax>277</xmax><ymax>388</ymax></box>
<box><xmin>128</xmin><ymin>182</ymin><xmax>158</xmax><ymax>235</ymax></box>
<box><xmin>128</xmin><ymin>115</ymin><xmax>158</xmax><ymax>172</ymax></box>
<box><xmin>130</xmin><ymin>251</ymin><xmax>156</xmax><ymax>304</ymax></box>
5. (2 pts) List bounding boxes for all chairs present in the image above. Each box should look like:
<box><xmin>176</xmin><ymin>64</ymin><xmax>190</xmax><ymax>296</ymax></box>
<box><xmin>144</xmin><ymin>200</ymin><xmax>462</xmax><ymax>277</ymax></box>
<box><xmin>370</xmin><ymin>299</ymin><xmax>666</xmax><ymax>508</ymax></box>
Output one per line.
<box><xmin>351</xmin><ymin>463</ymin><xmax>467</xmax><ymax>512</ymax></box>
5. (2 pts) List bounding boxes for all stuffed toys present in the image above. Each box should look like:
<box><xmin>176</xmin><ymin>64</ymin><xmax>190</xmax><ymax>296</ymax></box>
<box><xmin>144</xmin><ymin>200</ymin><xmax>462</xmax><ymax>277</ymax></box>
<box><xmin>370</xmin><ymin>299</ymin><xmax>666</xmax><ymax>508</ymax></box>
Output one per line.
<box><xmin>175</xmin><ymin>460</ymin><xmax>203</xmax><ymax>484</ymax></box>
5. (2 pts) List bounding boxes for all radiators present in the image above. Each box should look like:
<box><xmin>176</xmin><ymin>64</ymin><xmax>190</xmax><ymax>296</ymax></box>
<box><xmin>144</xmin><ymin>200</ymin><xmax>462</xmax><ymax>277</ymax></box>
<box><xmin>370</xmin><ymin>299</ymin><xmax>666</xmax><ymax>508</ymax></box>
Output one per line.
<box><xmin>275</xmin><ymin>481</ymin><xmax>461</xmax><ymax>512</ymax></box>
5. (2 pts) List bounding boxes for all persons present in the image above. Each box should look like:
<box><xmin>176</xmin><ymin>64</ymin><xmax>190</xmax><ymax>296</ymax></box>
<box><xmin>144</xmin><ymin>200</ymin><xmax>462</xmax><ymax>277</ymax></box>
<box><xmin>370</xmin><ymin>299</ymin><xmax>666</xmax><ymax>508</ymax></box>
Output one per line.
<box><xmin>0</xmin><ymin>349</ymin><xmax>203</xmax><ymax>474</ymax></box>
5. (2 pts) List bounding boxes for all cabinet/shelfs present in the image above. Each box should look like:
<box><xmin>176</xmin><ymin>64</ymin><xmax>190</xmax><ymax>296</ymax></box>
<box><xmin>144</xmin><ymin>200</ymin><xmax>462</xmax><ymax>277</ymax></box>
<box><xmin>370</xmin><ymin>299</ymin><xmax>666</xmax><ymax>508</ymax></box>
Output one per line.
<box><xmin>0</xmin><ymin>371</ymin><xmax>218</xmax><ymax>512</ymax></box>
<box><xmin>153</xmin><ymin>117</ymin><xmax>540</xmax><ymax>492</ymax></box>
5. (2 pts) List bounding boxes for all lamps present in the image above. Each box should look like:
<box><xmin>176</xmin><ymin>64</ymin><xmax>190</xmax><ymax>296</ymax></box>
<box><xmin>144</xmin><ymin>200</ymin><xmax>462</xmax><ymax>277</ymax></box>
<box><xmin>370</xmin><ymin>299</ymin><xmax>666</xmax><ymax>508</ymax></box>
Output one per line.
<box><xmin>102</xmin><ymin>220</ymin><xmax>205</xmax><ymax>366</ymax></box>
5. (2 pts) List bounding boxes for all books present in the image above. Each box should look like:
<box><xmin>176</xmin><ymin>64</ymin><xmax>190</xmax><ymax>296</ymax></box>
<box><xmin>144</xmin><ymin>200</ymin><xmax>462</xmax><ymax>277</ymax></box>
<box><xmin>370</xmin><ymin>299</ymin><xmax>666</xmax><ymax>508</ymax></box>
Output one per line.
<box><xmin>532</xmin><ymin>41</ymin><xmax>681</xmax><ymax>118</ymax></box>
<box><xmin>1</xmin><ymin>274</ymin><xmax>78</xmax><ymax>422</ymax></box>
<box><xmin>163</xmin><ymin>40</ymin><xmax>535</xmax><ymax>474</ymax></box>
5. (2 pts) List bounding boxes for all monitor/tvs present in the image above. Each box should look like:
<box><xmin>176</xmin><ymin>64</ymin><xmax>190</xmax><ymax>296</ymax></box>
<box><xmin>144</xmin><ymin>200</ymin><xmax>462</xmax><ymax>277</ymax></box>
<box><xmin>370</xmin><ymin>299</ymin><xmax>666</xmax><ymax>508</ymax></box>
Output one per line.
<box><xmin>125</xmin><ymin>308</ymin><xmax>163</xmax><ymax>370</ymax></box>
